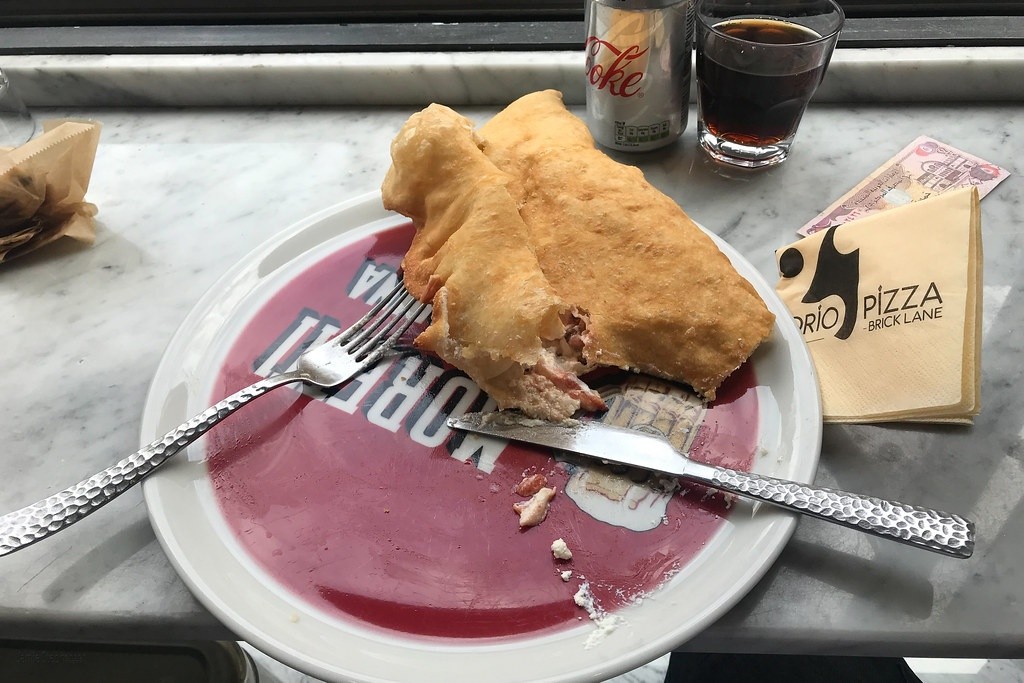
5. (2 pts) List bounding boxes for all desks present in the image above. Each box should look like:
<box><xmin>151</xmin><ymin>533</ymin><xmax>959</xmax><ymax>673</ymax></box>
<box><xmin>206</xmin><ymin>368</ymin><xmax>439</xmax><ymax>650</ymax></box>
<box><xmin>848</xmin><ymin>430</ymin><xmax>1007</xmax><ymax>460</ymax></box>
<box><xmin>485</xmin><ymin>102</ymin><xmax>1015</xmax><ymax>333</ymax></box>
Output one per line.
<box><xmin>0</xmin><ymin>50</ymin><xmax>1023</xmax><ymax>683</ymax></box>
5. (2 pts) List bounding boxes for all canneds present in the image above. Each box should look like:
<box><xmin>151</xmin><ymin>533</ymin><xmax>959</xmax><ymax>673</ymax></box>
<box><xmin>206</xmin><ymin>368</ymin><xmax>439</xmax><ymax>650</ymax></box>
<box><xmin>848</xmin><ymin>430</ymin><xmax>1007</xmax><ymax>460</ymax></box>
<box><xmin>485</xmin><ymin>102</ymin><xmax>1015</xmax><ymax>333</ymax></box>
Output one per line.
<box><xmin>583</xmin><ymin>0</ymin><xmax>694</xmax><ymax>154</ymax></box>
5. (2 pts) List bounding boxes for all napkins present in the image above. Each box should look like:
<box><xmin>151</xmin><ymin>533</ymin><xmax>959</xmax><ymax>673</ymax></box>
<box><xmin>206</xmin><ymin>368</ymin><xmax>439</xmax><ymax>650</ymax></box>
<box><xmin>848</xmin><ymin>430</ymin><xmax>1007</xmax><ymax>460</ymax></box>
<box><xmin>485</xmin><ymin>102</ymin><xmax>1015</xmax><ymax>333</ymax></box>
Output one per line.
<box><xmin>770</xmin><ymin>185</ymin><xmax>981</xmax><ymax>423</ymax></box>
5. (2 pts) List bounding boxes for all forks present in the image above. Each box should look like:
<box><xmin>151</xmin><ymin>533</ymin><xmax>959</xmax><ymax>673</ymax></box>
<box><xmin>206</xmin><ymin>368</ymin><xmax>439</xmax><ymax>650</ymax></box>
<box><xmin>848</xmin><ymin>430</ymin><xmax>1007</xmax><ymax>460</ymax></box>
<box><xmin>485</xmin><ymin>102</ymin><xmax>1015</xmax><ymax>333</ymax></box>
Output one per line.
<box><xmin>0</xmin><ymin>280</ymin><xmax>427</xmax><ymax>557</ymax></box>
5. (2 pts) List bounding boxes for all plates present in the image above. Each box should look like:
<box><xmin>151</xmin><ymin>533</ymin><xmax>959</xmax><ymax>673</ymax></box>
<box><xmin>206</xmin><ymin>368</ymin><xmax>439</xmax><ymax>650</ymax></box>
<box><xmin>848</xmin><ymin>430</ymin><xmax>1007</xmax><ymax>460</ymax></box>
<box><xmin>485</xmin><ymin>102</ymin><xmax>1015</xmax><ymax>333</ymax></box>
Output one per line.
<box><xmin>141</xmin><ymin>190</ymin><xmax>822</xmax><ymax>683</ymax></box>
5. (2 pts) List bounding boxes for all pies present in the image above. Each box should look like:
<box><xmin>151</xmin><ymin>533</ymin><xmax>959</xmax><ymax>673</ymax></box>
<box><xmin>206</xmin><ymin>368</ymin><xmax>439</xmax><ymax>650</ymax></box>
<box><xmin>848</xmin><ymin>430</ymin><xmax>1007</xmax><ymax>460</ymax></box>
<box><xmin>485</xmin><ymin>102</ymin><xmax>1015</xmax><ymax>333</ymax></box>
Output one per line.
<box><xmin>382</xmin><ymin>87</ymin><xmax>777</xmax><ymax>427</ymax></box>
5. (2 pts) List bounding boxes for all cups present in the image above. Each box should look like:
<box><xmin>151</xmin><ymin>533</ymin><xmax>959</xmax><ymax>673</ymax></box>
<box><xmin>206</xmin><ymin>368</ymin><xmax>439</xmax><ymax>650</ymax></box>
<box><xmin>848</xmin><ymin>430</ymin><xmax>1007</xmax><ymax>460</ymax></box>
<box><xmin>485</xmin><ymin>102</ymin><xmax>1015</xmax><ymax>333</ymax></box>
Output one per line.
<box><xmin>696</xmin><ymin>0</ymin><xmax>845</xmax><ymax>167</ymax></box>
<box><xmin>0</xmin><ymin>68</ymin><xmax>35</xmax><ymax>150</ymax></box>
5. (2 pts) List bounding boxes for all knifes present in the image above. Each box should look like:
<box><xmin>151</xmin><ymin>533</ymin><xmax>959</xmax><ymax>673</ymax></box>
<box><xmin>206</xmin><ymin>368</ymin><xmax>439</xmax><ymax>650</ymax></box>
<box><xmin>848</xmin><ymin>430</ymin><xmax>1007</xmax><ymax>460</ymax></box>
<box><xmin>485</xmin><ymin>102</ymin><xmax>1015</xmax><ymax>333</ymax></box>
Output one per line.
<box><xmin>447</xmin><ymin>411</ymin><xmax>976</xmax><ymax>559</ymax></box>
